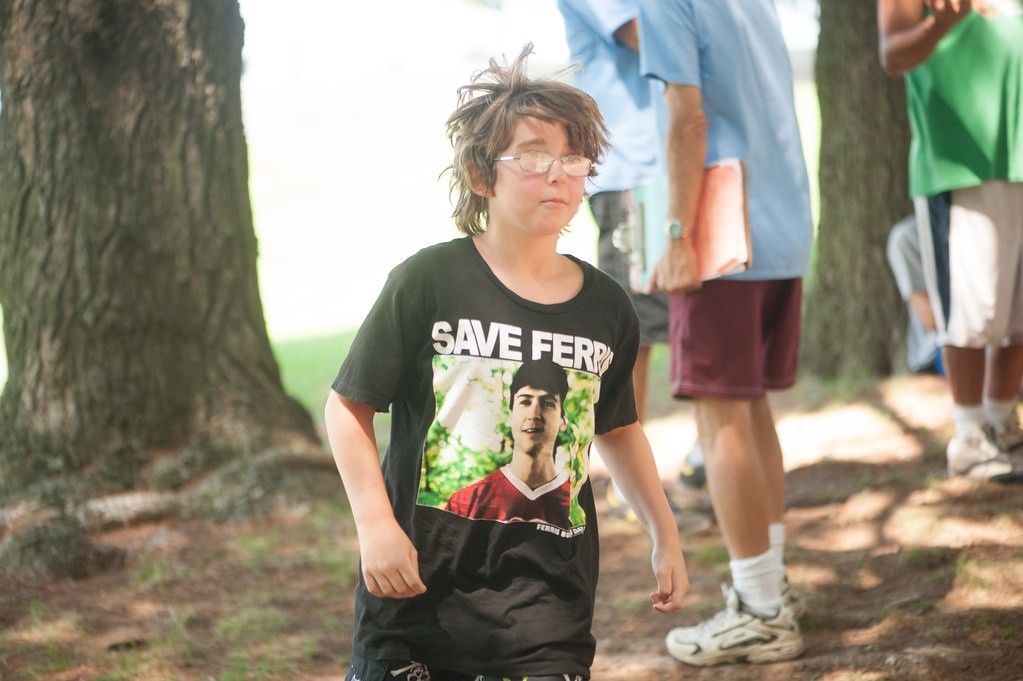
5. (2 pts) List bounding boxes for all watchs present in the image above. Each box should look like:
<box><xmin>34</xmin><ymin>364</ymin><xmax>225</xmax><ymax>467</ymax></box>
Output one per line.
<box><xmin>663</xmin><ymin>223</ymin><xmax>690</xmax><ymax>239</ymax></box>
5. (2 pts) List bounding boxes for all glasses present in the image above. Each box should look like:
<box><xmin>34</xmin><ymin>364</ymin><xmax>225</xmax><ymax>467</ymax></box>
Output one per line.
<box><xmin>490</xmin><ymin>150</ymin><xmax>596</xmax><ymax>177</ymax></box>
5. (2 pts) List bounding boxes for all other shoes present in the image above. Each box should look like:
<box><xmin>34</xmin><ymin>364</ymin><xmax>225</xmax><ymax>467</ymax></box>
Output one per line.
<box><xmin>980</xmin><ymin>403</ymin><xmax>1023</xmax><ymax>450</ymax></box>
<box><xmin>945</xmin><ymin>428</ymin><xmax>1022</xmax><ymax>480</ymax></box>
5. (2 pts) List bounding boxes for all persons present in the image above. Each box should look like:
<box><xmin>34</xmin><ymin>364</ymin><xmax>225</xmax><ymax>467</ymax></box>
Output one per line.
<box><xmin>876</xmin><ymin>1</ymin><xmax>1023</xmax><ymax>480</ymax></box>
<box><xmin>323</xmin><ymin>43</ymin><xmax>690</xmax><ymax>681</ymax></box>
<box><xmin>558</xmin><ymin>0</ymin><xmax>813</xmax><ymax>668</ymax></box>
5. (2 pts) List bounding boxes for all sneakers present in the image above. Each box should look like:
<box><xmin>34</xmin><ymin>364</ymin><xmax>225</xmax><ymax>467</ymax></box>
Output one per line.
<box><xmin>666</xmin><ymin>571</ymin><xmax>806</xmax><ymax>666</ymax></box>
